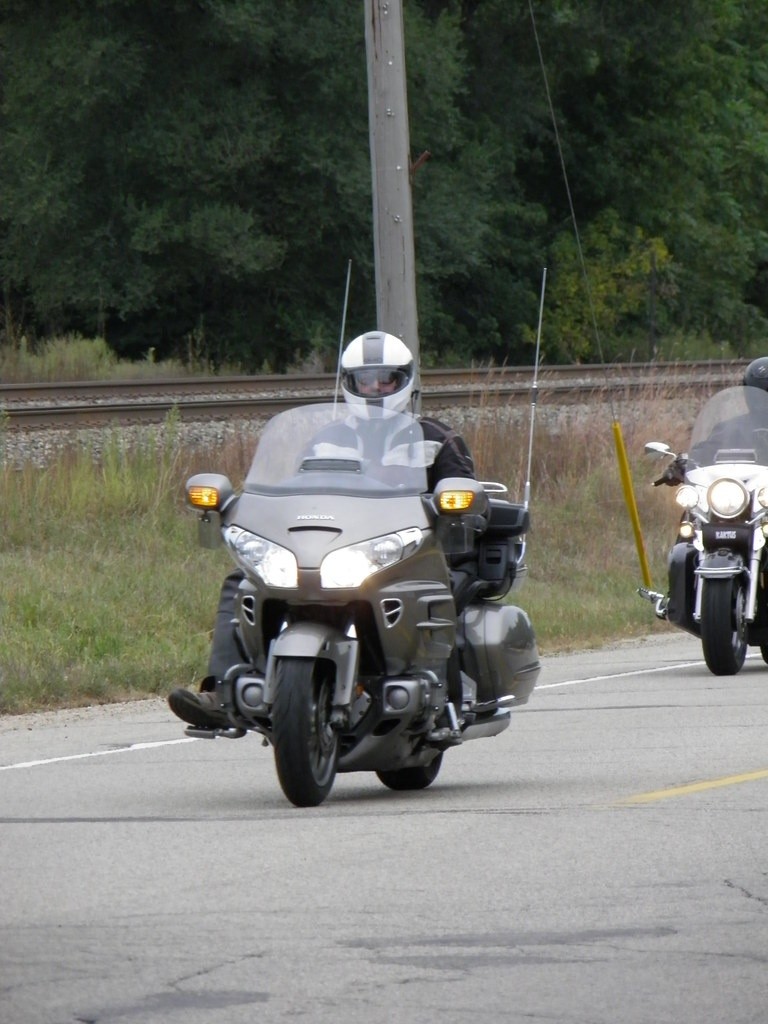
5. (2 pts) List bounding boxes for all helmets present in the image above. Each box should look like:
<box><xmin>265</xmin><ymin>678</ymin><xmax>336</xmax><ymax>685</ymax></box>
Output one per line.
<box><xmin>743</xmin><ymin>357</ymin><xmax>768</xmax><ymax>413</ymax></box>
<box><xmin>340</xmin><ymin>331</ymin><xmax>414</xmax><ymax>418</ymax></box>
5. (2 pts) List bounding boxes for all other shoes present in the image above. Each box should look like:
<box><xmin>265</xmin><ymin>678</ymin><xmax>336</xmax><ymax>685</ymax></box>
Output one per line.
<box><xmin>168</xmin><ymin>690</ymin><xmax>235</xmax><ymax>729</ymax></box>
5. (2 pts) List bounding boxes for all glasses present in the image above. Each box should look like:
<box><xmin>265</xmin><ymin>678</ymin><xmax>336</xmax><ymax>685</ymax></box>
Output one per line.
<box><xmin>351</xmin><ymin>371</ymin><xmax>396</xmax><ymax>386</ymax></box>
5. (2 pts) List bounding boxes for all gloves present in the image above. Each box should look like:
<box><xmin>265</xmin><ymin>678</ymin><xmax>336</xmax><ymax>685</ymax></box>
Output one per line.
<box><xmin>663</xmin><ymin>463</ymin><xmax>684</xmax><ymax>486</ymax></box>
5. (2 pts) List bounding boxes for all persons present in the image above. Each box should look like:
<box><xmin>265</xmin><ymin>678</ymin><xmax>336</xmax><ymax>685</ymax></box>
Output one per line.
<box><xmin>662</xmin><ymin>357</ymin><xmax>768</xmax><ymax>487</ymax></box>
<box><xmin>168</xmin><ymin>331</ymin><xmax>491</xmax><ymax>729</ymax></box>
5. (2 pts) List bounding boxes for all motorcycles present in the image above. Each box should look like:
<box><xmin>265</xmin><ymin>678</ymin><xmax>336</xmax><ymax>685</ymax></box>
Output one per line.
<box><xmin>635</xmin><ymin>384</ymin><xmax>768</xmax><ymax>678</ymax></box>
<box><xmin>181</xmin><ymin>260</ymin><xmax>543</xmax><ymax>812</ymax></box>
